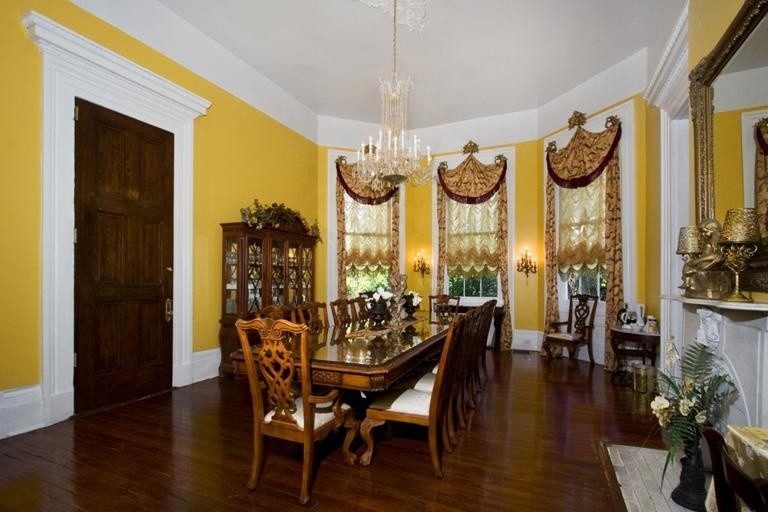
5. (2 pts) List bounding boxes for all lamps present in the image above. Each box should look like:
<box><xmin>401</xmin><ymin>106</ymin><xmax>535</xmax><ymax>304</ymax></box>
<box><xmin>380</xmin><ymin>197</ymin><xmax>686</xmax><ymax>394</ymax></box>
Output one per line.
<box><xmin>354</xmin><ymin>0</ymin><xmax>431</xmax><ymax>190</ymax></box>
<box><xmin>413</xmin><ymin>249</ymin><xmax>430</xmax><ymax>278</ymax></box>
<box><xmin>517</xmin><ymin>247</ymin><xmax>536</xmax><ymax>277</ymax></box>
<box><xmin>676</xmin><ymin>226</ymin><xmax>699</xmax><ymax>296</ymax></box>
<box><xmin>718</xmin><ymin>208</ymin><xmax>761</xmax><ymax>302</ymax></box>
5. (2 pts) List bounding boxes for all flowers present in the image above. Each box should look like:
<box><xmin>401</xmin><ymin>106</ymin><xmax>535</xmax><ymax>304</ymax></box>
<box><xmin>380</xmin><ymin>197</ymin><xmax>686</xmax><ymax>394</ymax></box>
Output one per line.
<box><xmin>637</xmin><ymin>341</ymin><xmax>739</xmax><ymax>488</ymax></box>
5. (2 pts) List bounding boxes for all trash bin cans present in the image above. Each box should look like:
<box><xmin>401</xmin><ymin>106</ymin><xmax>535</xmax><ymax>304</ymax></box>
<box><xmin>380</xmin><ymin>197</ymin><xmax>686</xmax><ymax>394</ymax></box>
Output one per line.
<box><xmin>634</xmin><ymin>365</ymin><xmax>656</xmax><ymax>392</ymax></box>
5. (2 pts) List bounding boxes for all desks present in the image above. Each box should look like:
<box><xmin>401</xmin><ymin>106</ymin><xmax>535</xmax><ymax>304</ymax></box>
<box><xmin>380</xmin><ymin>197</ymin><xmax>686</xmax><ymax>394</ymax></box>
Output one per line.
<box><xmin>610</xmin><ymin>328</ymin><xmax>659</xmax><ymax>386</ymax></box>
<box><xmin>596</xmin><ymin>440</ymin><xmax>752</xmax><ymax>512</ymax></box>
<box><xmin>438</xmin><ymin>304</ymin><xmax>502</xmax><ymax>351</ymax></box>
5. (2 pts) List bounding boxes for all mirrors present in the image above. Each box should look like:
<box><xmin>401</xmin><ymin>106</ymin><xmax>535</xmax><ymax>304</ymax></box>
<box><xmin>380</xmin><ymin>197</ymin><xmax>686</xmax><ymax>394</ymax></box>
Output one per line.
<box><xmin>688</xmin><ymin>0</ymin><xmax>768</xmax><ymax>293</ymax></box>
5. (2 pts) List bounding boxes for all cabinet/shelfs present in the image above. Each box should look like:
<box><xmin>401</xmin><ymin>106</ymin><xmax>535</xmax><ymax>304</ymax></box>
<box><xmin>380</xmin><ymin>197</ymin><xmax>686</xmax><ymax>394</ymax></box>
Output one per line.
<box><xmin>220</xmin><ymin>224</ymin><xmax>317</xmax><ymax>378</ymax></box>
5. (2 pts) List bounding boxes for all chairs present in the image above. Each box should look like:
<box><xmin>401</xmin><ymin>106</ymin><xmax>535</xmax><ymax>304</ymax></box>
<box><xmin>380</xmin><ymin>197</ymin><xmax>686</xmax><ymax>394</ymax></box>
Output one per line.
<box><xmin>543</xmin><ymin>294</ymin><xmax>598</xmax><ymax>368</ymax></box>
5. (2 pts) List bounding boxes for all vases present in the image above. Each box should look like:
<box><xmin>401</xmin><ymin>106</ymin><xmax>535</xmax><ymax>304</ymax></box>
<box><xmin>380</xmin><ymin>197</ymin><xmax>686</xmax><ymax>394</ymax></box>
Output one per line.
<box><xmin>670</xmin><ymin>436</ymin><xmax>707</xmax><ymax>512</ymax></box>
